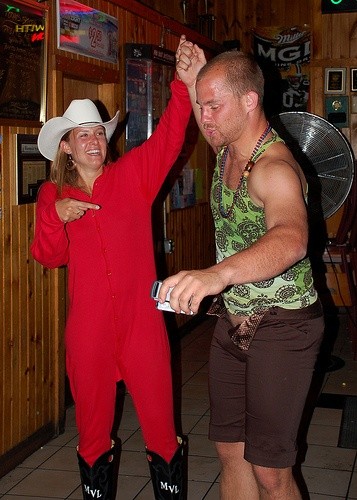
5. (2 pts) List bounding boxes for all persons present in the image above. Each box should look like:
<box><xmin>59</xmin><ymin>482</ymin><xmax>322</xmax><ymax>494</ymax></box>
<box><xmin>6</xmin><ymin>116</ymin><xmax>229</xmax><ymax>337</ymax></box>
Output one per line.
<box><xmin>32</xmin><ymin>34</ymin><xmax>199</xmax><ymax>500</ymax></box>
<box><xmin>158</xmin><ymin>39</ymin><xmax>330</xmax><ymax>500</ymax></box>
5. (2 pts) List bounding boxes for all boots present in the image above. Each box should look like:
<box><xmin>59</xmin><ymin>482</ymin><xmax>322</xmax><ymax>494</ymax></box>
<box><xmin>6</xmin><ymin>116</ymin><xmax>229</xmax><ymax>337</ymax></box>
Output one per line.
<box><xmin>145</xmin><ymin>435</ymin><xmax>185</xmax><ymax>500</ymax></box>
<box><xmin>76</xmin><ymin>439</ymin><xmax>116</xmax><ymax>500</ymax></box>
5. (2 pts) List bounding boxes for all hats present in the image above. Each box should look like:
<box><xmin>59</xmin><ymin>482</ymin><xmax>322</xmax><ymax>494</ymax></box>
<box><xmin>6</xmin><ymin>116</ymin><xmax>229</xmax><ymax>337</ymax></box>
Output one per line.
<box><xmin>37</xmin><ymin>99</ymin><xmax>120</xmax><ymax>161</ymax></box>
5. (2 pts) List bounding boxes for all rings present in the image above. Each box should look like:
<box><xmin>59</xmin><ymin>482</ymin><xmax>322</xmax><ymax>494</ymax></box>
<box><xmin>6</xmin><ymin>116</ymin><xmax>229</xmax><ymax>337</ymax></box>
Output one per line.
<box><xmin>75</xmin><ymin>210</ymin><xmax>81</xmax><ymax>214</ymax></box>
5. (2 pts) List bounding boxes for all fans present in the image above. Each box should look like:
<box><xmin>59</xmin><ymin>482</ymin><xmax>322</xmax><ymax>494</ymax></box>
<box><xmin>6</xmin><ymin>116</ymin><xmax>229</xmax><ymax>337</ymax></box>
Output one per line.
<box><xmin>269</xmin><ymin>112</ymin><xmax>354</xmax><ymax>222</ymax></box>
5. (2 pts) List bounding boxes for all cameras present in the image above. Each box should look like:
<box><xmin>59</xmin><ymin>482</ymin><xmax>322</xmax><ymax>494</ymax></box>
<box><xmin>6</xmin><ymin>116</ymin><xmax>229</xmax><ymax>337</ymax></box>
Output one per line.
<box><xmin>151</xmin><ymin>282</ymin><xmax>194</xmax><ymax>315</ymax></box>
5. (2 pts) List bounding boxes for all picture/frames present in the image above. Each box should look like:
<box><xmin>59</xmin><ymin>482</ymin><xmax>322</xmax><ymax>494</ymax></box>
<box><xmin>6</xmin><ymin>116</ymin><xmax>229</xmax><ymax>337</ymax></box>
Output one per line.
<box><xmin>0</xmin><ymin>0</ymin><xmax>51</xmax><ymax>128</ymax></box>
<box><xmin>324</xmin><ymin>67</ymin><xmax>347</xmax><ymax>94</ymax></box>
<box><xmin>350</xmin><ymin>68</ymin><xmax>357</xmax><ymax>92</ymax></box>
<box><xmin>350</xmin><ymin>94</ymin><xmax>357</xmax><ymax>114</ymax></box>
<box><xmin>15</xmin><ymin>133</ymin><xmax>50</xmax><ymax>206</ymax></box>
<box><xmin>324</xmin><ymin>95</ymin><xmax>350</xmax><ymax>128</ymax></box>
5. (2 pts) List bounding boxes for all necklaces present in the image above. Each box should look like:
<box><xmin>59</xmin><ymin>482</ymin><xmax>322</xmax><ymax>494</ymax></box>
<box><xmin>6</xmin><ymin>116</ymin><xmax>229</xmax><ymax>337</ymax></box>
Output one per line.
<box><xmin>218</xmin><ymin>122</ymin><xmax>274</xmax><ymax>219</ymax></box>
